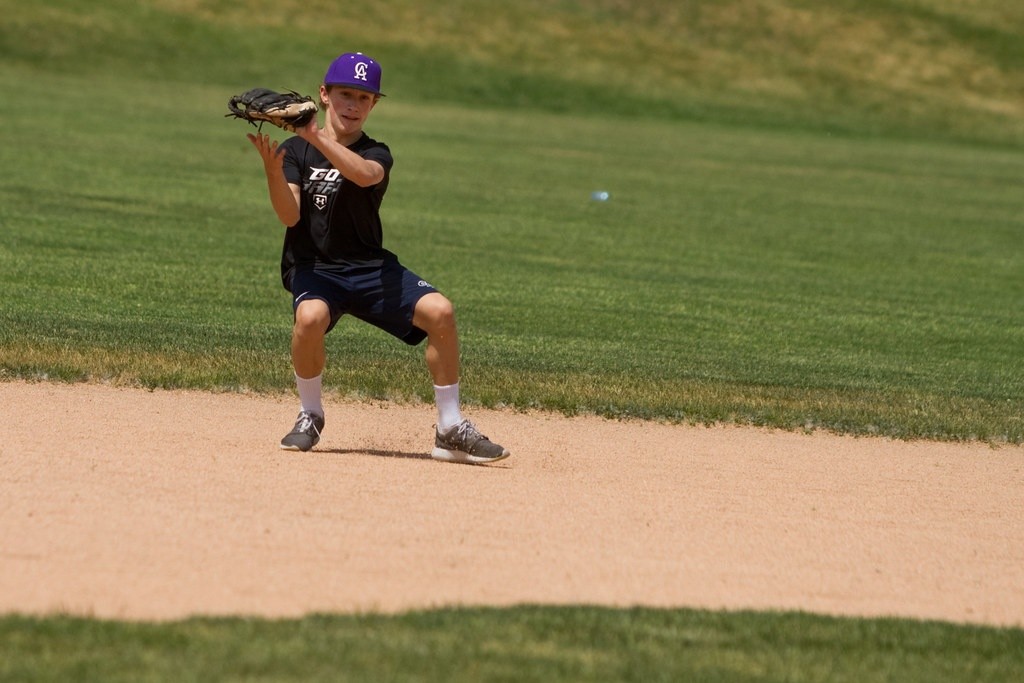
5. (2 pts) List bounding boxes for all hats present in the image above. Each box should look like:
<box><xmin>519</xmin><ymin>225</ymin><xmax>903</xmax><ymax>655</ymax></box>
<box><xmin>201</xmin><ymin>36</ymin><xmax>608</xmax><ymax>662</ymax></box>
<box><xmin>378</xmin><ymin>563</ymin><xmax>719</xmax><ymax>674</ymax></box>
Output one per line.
<box><xmin>324</xmin><ymin>51</ymin><xmax>387</xmax><ymax>96</ymax></box>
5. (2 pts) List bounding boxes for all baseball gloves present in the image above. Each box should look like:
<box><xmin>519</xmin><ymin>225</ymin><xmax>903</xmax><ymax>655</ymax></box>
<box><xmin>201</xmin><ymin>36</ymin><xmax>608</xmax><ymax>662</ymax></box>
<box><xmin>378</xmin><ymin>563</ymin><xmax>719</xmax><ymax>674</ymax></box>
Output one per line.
<box><xmin>227</xmin><ymin>88</ymin><xmax>318</xmax><ymax>129</ymax></box>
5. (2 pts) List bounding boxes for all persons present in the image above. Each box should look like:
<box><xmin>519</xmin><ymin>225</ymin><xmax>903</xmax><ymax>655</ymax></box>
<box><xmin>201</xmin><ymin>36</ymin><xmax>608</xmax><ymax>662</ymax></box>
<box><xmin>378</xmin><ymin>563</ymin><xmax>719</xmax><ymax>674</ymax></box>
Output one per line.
<box><xmin>246</xmin><ymin>51</ymin><xmax>511</xmax><ymax>462</ymax></box>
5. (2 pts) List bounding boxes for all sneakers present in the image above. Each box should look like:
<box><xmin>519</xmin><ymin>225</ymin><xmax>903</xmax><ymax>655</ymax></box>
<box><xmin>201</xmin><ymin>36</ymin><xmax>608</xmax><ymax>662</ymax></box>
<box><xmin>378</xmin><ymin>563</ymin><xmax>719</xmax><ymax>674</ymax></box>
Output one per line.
<box><xmin>431</xmin><ymin>419</ymin><xmax>510</xmax><ymax>462</ymax></box>
<box><xmin>280</xmin><ymin>409</ymin><xmax>325</xmax><ymax>451</ymax></box>
